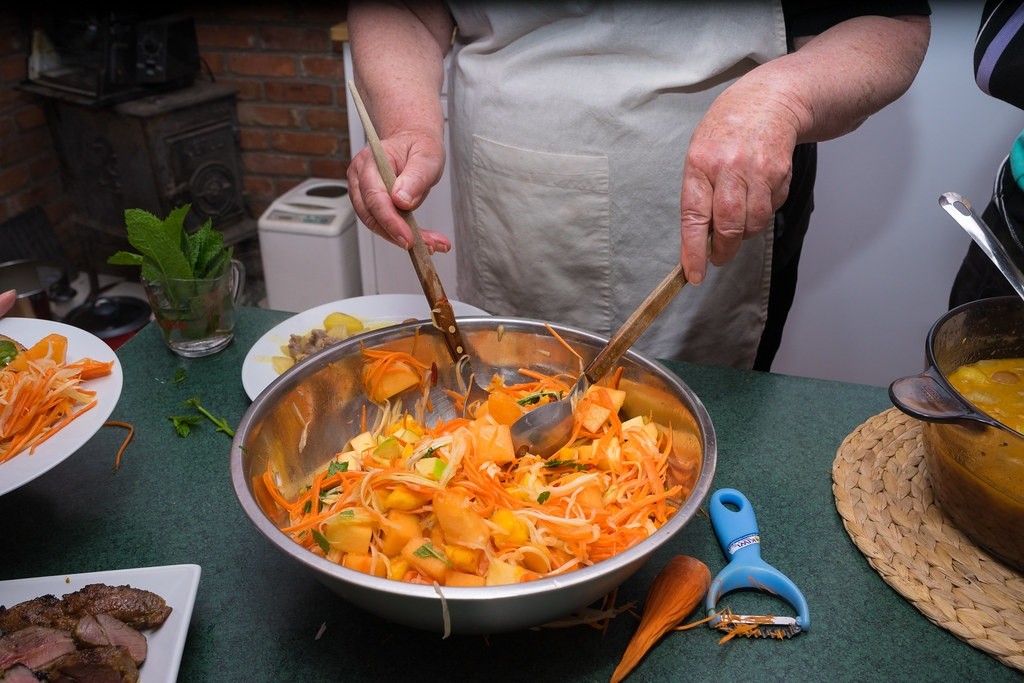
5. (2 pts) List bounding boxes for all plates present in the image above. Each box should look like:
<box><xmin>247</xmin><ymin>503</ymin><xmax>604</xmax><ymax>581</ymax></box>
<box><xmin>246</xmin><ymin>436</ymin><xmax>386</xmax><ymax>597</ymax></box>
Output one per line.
<box><xmin>0</xmin><ymin>314</ymin><xmax>124</xmax><ymax>497</ymax></box>
<box><xmin>241</xmin><ymin>292</ymin><xmax>494</xmax><ymax>403</ymax></box>
<box><xmin>0</xmin><ymin>563</ymin><xmax>202</xmax><ymax>683</ymax></box>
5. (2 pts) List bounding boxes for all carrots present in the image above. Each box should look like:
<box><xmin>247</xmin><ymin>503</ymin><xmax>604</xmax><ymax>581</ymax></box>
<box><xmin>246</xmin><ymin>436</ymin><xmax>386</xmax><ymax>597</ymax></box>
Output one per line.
<box><xmin>0</xmin><ymin>352</ymin><xmax>134</xmax><ymax>470</ymax></box>
<box><xmin>257</xmin><ymin>323</ymin><xmax>683</xmax><ymax>587</ymax></box>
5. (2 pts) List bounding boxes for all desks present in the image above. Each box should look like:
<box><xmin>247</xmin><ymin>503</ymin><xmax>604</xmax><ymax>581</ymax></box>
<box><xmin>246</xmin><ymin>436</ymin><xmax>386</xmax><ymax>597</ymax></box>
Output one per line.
<box><xmin>0</xmin><ymin>308</ymin><xmax>1023</xmax><ymax>683</ymax></box>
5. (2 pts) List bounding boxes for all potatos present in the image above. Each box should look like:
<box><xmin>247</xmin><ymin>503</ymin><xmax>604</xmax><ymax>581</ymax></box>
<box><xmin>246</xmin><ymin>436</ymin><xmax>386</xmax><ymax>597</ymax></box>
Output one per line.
<box><xmin>271</xmin><ymin>311</ymin><xmax>364</xmax><ymax>375</ymax></box>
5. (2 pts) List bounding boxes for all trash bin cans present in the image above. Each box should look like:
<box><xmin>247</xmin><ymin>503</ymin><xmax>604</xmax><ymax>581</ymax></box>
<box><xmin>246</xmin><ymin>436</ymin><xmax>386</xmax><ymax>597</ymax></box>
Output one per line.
<box><xmin>257</xmin><ymin>176</ymin><xmax>360</xmax><ymax>312</ymax></box>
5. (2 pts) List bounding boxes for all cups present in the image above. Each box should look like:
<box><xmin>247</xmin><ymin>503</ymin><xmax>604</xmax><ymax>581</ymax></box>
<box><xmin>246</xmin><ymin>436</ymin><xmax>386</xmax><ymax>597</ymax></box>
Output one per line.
<box><xmin>139</xmin><ymin>264</ymin><xmax>237</xmax><ymax>358</ymax></box>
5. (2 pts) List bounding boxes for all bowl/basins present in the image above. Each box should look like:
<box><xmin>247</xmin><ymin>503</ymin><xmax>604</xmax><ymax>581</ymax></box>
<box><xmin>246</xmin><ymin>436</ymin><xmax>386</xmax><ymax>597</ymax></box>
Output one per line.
<box><xmin>888</xmin><ymin>294</ymin><xmax>1024</xmax><ymax>578</ymax></box>
<box><xmin>229</xmin><ymin>316</ymin><xmax>718</xmax><ymax>638</ymax></box>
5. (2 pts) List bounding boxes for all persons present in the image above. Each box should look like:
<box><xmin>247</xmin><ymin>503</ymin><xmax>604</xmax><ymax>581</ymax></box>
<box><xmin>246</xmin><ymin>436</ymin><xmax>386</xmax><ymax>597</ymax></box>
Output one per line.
<box><xmin>340</xmin><ymin>1</ymin><xmax>934</xmax><ymax>375</ymax></box>
<box><xmin>948</xmin><ymin>0</ymin><xmax>1024</xmax><ymax>311</ymax></box>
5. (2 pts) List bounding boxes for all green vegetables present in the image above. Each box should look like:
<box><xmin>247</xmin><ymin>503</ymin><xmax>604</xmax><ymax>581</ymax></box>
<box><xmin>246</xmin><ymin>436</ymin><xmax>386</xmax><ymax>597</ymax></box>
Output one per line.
<box><xmin>0</xmin><ymin>339</ymin><xmax>20</xmax><ymax>370</ymax></box>
<box><xmin>166</xmin><ymin>367</ymin><xmax>236</xmax><ymax>439</ymax></box>
